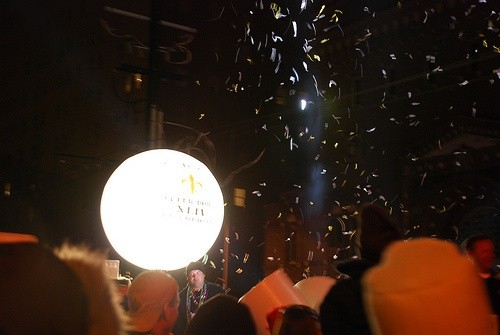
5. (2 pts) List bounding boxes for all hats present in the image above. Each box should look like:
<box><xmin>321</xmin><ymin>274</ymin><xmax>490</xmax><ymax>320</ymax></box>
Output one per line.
<box><xmin>336</xmin><ymin>229</ymin><xmax>374</xmax><ymax>277</ymax></box>
<box><xmin>359</xmin><ymin>236</ymin><xmax>500</xmax><ymax>335</ymax></box>
<box><xmin>185</xmin><ymin>261</ymin><xmax>206</xmax><ymax>273</ymax></box>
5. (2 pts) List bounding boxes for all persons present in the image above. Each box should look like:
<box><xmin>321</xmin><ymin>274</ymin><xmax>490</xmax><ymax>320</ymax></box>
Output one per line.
<box><xmin>0</xmin><ymin>235</ymin><xmax>497</xmax><ymax>335</ymax></box>
<box><xmin>173</xmin><ymin>261</ymin><xmax>224</xmax><ymax>334</ymax></box>
<box><xmin>459</xmin><ymin>236</ymin><xmax>500</xmax><ymax>315</ymax></box>
